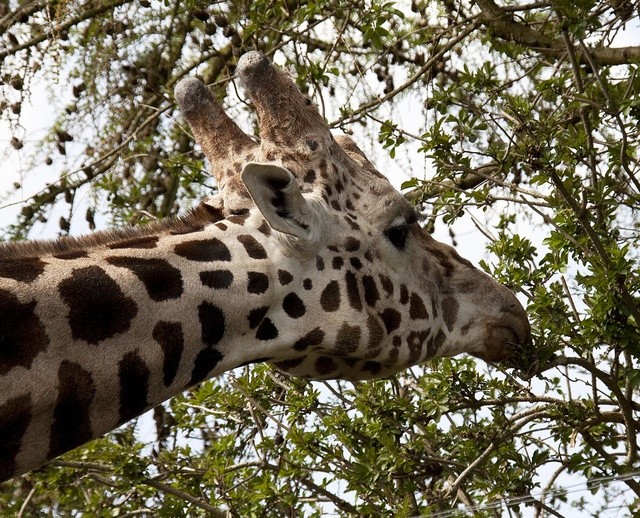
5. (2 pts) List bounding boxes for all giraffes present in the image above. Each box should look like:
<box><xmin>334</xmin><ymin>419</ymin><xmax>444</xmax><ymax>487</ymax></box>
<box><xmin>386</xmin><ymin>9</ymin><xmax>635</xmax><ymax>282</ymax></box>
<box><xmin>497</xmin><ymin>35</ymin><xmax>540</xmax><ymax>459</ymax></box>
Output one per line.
<box><xmin>1</xmin><ymin>51</ymin><xmax>530</xmax><ymax>483</ymax></box>
